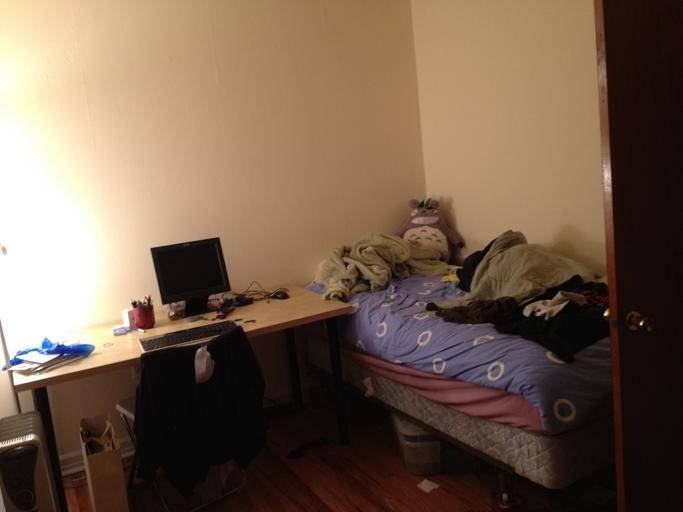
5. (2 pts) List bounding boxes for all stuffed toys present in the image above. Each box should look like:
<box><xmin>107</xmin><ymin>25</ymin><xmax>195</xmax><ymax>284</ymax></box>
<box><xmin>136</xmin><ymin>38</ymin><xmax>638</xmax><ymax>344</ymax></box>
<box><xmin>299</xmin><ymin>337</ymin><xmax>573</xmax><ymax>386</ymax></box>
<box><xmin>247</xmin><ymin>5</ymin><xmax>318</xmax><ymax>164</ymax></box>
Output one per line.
<box><xmin>396</xmin><ymin>200</ymin><xmax>466</xmax><ymax>269</ymax></box>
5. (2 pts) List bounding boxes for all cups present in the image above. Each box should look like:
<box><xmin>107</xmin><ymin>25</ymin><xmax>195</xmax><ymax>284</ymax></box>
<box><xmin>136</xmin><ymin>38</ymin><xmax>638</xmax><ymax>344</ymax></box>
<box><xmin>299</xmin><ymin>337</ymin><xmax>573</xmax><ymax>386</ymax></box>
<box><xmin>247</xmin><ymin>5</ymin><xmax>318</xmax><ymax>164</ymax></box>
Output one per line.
<box><xmin>133</xmin><ymin>307</ymin><xmax>155</xmax><ymax>329</ymax></box>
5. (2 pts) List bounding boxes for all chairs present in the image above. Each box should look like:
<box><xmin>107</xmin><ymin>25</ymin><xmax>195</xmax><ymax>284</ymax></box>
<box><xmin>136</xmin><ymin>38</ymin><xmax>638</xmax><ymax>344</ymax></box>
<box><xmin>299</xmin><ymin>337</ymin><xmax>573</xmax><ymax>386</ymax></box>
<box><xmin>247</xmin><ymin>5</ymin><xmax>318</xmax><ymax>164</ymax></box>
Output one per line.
<box><xmin>132</xmin><ymin>325</ymin><xmax>271</xmax><ymax>499</ymax></box>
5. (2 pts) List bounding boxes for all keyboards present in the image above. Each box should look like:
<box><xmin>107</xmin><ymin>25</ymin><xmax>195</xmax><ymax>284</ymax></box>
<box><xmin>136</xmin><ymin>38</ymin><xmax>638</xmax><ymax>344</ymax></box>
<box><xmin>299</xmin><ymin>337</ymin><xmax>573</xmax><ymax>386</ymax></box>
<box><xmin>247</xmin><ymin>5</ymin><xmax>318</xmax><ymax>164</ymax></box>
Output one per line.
<box><xmin>138</xmin><ymin>320</ymin><xmax>237</xmax><ymax>352</ymax></box>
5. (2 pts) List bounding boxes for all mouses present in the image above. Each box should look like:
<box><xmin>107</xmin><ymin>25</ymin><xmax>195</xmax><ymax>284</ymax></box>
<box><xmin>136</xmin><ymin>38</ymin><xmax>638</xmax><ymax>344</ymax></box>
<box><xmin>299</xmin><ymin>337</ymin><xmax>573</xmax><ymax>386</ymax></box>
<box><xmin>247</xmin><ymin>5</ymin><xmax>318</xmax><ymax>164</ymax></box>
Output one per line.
<box><xmin>272</xmin><ymin>290</ymin><xmax>288</xmax><ymax>299</ymax></box>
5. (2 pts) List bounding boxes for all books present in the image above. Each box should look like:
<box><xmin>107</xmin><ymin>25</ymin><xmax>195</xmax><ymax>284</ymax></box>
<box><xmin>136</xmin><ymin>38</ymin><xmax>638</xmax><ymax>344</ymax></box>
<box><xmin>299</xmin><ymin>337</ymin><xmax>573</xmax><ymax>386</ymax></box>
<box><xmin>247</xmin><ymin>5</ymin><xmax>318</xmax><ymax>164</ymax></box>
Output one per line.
<box><xmin>11</xmin><ymin>352</ymin><xmax>84</xmax><ymax>375</ymax></box>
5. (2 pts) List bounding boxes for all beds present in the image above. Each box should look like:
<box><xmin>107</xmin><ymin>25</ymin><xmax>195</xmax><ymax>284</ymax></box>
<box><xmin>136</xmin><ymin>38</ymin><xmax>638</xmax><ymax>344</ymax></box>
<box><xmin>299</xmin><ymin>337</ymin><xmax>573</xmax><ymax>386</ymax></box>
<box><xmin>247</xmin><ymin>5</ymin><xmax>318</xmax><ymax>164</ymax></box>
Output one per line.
<box><xmin>348</xmin><ymin>246</ymin><xmax>609</xmax><ymax>483</ymax></box>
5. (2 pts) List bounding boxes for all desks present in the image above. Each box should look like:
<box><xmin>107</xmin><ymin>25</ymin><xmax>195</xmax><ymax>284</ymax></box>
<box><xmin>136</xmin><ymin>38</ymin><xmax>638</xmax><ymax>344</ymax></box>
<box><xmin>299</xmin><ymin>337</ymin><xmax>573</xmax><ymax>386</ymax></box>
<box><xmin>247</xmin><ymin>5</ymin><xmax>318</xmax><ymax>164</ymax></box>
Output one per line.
<box><xmin>25</xmin><ymin>285</ymin><xmax>354</xmax><ymax>506</ymax></box>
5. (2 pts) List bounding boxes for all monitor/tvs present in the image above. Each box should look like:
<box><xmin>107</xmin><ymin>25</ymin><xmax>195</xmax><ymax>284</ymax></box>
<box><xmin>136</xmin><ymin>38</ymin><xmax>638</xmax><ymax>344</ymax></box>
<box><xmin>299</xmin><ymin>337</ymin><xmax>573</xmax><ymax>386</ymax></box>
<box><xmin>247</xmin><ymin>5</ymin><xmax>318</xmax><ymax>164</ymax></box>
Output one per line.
<box><xmin>150</xmin><ymin>237</ymin><xmax>231</xmax><ymax>320</ymax></box>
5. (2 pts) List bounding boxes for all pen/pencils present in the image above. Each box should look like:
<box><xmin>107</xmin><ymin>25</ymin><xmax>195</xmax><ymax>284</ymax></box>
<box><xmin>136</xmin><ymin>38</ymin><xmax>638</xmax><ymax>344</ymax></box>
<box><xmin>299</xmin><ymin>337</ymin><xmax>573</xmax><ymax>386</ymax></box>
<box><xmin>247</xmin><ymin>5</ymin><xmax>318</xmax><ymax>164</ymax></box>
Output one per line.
<box><xmin>137</xmin><ymin>328</ymin><xmax>145</xmax><ymax>333</ymax></box>
<box><xmin>130</xmin><ymin>294</ymin><xmax>151</xmax><ymax>309</ymax></box>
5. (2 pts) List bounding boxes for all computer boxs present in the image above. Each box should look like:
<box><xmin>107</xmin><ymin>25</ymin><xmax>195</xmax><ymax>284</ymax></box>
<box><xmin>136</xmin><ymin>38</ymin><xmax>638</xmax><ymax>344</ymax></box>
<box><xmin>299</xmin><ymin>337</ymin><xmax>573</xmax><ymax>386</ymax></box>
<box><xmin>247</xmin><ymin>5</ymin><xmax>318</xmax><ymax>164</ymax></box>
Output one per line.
<box><xmin>0</xmin><ymin>412</ymin><xmax>60</xmax><ymax>512</ymax></box>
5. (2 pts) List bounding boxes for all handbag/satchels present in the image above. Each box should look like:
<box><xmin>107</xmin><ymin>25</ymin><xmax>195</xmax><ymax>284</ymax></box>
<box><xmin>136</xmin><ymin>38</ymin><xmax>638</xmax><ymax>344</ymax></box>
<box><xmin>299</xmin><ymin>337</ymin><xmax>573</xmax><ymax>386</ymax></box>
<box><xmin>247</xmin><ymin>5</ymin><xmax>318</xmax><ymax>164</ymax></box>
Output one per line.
<box><xmin>79</xmin><ymin>412</ymin><xmax>130</xmax><ymax>512</ymax></box>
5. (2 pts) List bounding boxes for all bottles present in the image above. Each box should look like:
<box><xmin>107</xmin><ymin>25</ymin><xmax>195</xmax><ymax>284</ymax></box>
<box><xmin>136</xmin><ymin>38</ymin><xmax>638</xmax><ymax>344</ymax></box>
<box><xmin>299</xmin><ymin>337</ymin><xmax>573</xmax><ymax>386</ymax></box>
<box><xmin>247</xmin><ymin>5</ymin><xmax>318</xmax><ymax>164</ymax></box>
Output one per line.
<box><xmin>489</xmin><ymin>487</ymin><xmax>530</xmax><ymax>511</ymax></box>
